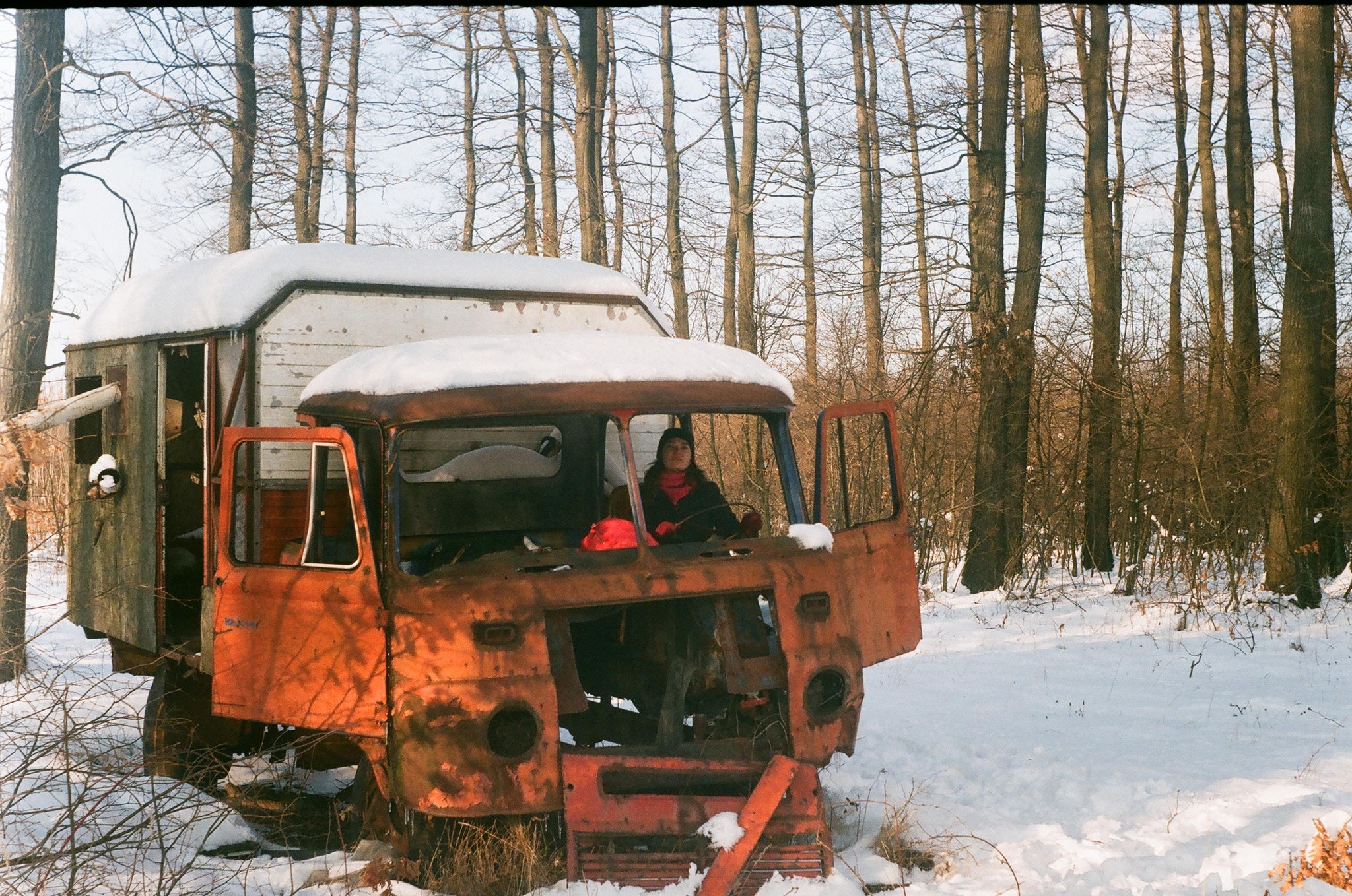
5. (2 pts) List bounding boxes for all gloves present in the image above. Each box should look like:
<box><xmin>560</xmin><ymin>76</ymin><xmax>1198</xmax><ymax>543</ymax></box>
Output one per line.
<box><xmin>655</xmin><ymin>522</ymin><xmax>676</xmax><ymax>538</ymax></box>
<box><xmin>741</xmin><ymin>513</ymin><xmax>762</xmax><ymax>535</ymax></box>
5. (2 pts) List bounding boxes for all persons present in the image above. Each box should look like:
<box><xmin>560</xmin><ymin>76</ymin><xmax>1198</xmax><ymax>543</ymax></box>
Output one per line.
<box><xmin>638</xmin><ymin>427</ymin><xmax>762</xmax><ymax>547</ymax></box>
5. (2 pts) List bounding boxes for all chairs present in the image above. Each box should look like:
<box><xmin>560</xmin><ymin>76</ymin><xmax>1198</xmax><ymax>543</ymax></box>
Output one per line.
<box><xmin>609</xmin><ymin>484</ymin><xmax>633</xmax><ymax>522</ymax></box>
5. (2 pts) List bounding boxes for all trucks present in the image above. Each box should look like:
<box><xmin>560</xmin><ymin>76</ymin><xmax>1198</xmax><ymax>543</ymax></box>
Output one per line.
<box><xmin>61</xmin><ymin>242</ymin><xmax>923</xmax><ymax>894</ymax></box>
